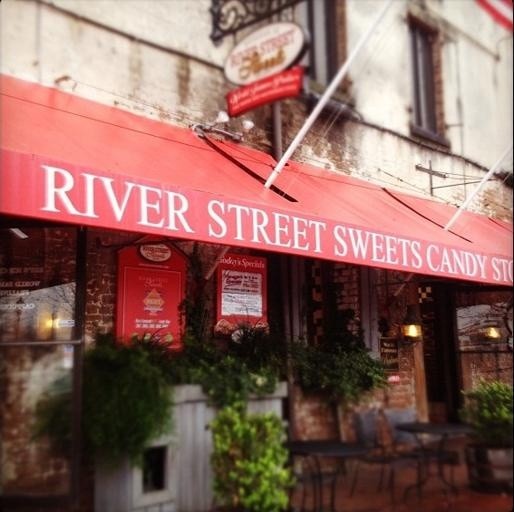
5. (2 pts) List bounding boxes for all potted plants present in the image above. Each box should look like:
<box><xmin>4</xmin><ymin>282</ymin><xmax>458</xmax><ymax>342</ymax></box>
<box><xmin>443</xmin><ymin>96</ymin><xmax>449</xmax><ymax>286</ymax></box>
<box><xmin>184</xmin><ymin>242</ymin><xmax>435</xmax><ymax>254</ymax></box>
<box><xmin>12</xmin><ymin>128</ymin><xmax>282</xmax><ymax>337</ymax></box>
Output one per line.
<box><xmin>457</xmin><ymin>378</ymin><xmax>514</xmax><ymax>494</ymax></box>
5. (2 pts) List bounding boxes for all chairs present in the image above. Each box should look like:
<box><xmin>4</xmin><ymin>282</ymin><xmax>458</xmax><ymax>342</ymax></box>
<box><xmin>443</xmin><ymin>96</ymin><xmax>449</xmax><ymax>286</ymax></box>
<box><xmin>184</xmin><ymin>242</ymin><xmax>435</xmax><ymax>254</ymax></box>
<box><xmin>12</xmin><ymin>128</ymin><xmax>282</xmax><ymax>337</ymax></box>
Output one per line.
<box><xmin>351</xmin><ymin>400</ymin><xmax>460</xmax><ymax>509</ymax></box>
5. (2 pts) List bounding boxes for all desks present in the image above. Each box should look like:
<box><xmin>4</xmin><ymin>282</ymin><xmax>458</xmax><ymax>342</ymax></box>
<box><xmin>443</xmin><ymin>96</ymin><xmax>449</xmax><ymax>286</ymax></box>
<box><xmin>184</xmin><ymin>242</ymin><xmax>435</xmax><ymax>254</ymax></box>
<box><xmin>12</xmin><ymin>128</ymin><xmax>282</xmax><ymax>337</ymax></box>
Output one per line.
<box><xmin>290</xmin><ymin>438</ymin><xmax>369</xmax><ymax>512</ymax></box>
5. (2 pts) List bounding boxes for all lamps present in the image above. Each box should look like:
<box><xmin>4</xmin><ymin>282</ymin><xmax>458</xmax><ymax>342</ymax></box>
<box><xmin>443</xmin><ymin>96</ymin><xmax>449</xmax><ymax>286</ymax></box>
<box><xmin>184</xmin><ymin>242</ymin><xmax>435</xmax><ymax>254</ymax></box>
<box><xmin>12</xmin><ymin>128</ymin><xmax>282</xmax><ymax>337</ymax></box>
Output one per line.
<box><xmin>477</xmin><ymin>313</ymin><xmax>507</xmax><ymax>343</ymax></box>
<box><xmin>400</xmin><ymin>302</ymin><xmax>425</xmax><ymax>348</ymax></box>
